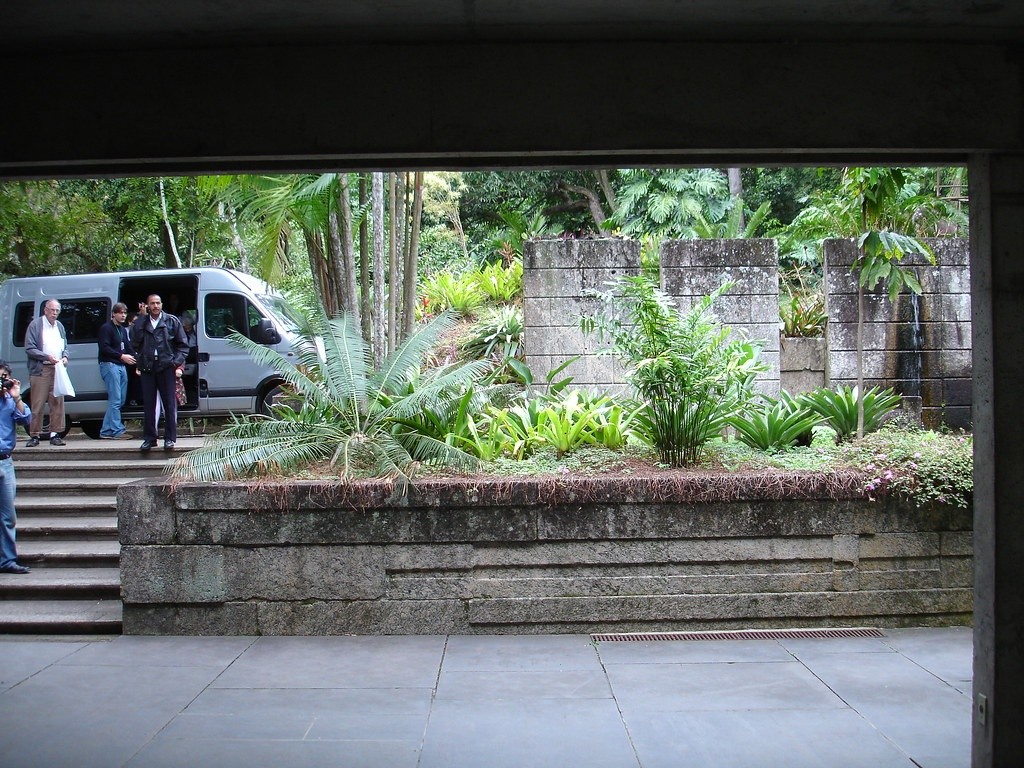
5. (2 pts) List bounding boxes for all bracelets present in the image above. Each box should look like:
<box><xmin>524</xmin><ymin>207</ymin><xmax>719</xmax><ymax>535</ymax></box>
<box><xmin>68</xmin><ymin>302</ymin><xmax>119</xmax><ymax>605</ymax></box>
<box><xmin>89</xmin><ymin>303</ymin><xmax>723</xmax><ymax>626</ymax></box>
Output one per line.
<box><xmin>15</xmin><ymin>396</ymin><xmax>22</xmax><ymax>403</ymax></box>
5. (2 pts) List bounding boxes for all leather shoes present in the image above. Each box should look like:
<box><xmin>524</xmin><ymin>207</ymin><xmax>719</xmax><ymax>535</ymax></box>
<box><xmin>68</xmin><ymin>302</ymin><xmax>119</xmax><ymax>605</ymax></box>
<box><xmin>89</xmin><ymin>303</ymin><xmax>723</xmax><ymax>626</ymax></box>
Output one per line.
<box><xmin>140</xmin><ymin>439</ymin><xmax>157</xmax><ymax>450</ymax></box>
<box><xmin>164</xmin><ymin>438</ymin><xmax>175</xmax><ymax>449</ymax></box>
<box><xmin>3</xmin><ymin>562</ymin><xmax>31</xmax><ymax>573</ymax></box>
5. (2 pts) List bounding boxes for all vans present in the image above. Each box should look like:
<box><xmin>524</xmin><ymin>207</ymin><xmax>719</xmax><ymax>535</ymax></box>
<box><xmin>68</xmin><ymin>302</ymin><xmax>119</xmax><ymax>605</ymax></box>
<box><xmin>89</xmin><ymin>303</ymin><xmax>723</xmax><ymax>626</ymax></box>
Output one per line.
<box><xmin>0</xmin><ymin>264</ymin><xmax>317</xmax><ymax>441</ymax></box>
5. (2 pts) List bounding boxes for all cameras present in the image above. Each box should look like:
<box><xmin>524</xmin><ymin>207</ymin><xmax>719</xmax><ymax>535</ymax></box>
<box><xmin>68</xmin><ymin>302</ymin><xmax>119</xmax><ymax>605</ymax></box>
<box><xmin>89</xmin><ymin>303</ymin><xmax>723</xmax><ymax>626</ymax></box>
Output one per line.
<box><xmin>0</xmin><ymin>373</ymin><xmax>14</xmax><ymax>392</ymax></box>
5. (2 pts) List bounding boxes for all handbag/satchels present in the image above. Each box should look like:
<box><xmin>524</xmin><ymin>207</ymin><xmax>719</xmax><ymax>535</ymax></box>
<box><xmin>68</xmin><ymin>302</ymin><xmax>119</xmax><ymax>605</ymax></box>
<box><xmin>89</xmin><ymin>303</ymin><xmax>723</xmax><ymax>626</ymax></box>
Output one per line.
<box><xmin>53</xmin><ymin>359</ymin><xmax>75</xmax><ymax>397</ymax></box>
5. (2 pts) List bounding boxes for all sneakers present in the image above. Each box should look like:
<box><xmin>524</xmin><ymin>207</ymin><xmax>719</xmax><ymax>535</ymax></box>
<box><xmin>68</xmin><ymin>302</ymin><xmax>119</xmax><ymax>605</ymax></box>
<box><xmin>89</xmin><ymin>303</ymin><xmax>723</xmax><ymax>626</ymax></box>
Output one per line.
<box><xmin>50</xmin><ymin>435</ymin><xmax>66</xmax><ymax>446</ymax></box>
<box><xmin>26</xmin><ymin>435</ymin><xmax>39</xmax><ymax>447</ymax></box>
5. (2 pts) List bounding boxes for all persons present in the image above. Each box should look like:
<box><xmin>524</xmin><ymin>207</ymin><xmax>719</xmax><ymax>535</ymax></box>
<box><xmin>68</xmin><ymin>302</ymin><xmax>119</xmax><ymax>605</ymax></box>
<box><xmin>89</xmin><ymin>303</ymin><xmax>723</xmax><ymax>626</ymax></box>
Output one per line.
<box><xmin>125</xmin><ymin>301</ymin><xmax>196</xmax><ymax>437</ymax></box>
<box><xmin>0</xmin><ymin>359</ymin><xmax>31</xmax><ymax>574</ymax></box>
<box><xmin>98</xmin><ymin>302</ymin><xmax>135</xmax><ymax>440</ymax></box>
<box><xmin>24</xmin><ymin>299</ymin><xmax>69</xmax><ymax>448</ymax></box>
<box><xmin>129</xmin><ymin>294</ymin><xmax>188</xmax><ymax>450</ymax></box>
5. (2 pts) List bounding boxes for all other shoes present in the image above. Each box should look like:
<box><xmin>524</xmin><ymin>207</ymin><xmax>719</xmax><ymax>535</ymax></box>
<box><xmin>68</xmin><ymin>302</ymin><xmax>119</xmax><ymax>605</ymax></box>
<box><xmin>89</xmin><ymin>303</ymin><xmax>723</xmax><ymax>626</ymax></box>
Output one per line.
<box><xmin>100</xmin><ymin>433</ymin><xmax>112</xmax><ymax>438</ymax></box>
<box><xmin>113</xmin><ymin>433</ymin><xmax>134</xmax><ymax>439</ymax></box>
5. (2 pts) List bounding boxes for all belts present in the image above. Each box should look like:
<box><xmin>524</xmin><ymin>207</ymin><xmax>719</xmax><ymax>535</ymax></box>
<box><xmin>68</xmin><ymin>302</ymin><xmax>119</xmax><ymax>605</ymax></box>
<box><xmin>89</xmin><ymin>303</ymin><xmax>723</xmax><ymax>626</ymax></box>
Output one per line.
<box><xmin>0</xmin><ymin>454</ymin><xmax>11</xmax><ymax>460</ymax></box>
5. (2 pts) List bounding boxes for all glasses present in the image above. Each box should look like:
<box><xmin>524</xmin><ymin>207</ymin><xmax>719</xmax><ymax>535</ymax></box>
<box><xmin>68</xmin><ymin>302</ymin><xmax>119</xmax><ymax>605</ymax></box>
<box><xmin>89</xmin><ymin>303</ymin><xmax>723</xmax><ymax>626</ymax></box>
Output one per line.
<box><xmin>45</xmin><ymin>306</ymin><xmax>60</xmax><ymax>314</ymax></box>
